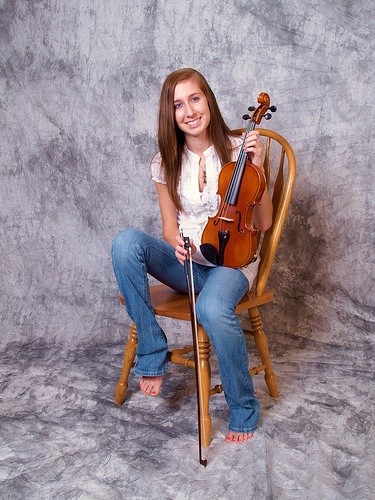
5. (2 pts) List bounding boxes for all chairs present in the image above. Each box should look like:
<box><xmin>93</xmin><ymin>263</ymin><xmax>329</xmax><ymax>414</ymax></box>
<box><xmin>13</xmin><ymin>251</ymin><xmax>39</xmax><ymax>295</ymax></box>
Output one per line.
<box><xmin>115</xmin><ymin>128</ymin><xmax>296</xmax><ymax>447</ymax></box>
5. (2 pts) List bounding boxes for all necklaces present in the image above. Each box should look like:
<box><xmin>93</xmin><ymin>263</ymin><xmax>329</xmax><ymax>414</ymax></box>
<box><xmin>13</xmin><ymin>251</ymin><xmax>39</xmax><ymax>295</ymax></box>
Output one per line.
<box><xmin>199</xmin><ymin>161</ymin><xmax>207</xmax><ymax>183</ymax></box>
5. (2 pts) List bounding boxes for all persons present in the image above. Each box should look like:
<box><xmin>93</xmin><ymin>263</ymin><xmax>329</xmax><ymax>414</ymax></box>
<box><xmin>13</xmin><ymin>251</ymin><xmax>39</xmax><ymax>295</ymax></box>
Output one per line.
<box><xmin>111</xmin><ymin>68</ymin><xmax>272</xmax><ymax>442</ymax></box>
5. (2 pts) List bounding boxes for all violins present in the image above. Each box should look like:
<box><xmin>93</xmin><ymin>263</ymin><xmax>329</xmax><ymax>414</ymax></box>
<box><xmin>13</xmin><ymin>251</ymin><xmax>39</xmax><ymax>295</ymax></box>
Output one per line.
<box><xmin>200</xmin><ymin>92</ymin><xmax>277</xmax><ymax>268</ymax></box>
